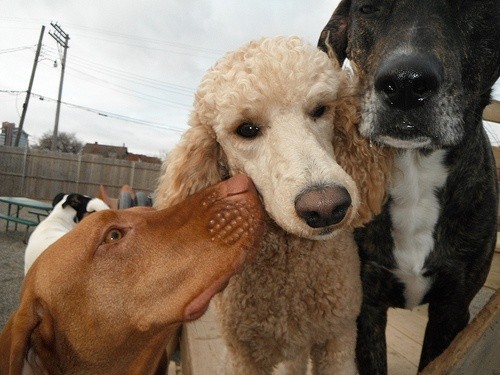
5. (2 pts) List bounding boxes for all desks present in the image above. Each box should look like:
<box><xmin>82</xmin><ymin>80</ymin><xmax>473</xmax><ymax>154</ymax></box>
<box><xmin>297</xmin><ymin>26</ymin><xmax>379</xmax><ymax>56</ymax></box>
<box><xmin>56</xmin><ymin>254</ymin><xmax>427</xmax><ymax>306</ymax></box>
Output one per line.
<box><xmin>0</xmin><ymin>196</ymin><xmax>54</xmax><ymax>232</ymax></box>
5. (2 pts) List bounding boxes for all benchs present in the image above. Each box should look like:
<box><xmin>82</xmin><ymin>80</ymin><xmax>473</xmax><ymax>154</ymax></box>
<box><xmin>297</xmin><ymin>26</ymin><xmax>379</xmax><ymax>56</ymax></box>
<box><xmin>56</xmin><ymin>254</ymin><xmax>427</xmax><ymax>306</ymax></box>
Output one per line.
<box><xmin>0</xmin><ymin>214</ymin><xmax>39</xmax><ymax>243</ymax></box>
<box><xmin>28</xmin><ymin>210</ymin><xmax>52</xmax><ymax>222</ymax></box>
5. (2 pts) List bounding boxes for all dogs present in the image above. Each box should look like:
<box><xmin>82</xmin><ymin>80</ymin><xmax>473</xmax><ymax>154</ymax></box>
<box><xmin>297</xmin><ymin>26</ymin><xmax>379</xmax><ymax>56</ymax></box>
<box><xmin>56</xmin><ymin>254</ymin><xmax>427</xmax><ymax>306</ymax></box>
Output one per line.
<box><xmin>25</xmin><ymin>190</ymin><xmax>110</xmax><ymax>278</ymax></box>
<box><xmin>0</xmin><ymin>173</ymin><xmax>264</xmax><ymax>375</ymax></box>
<box><xmin>151</xmin><ymin>36</ymin><xmax>406</xmax><ymax>375</ymax></box>
<box><xmin>316</xmin><ymin>0</ymin><xmax>500</xmax><ymax>375</ymax></box>
